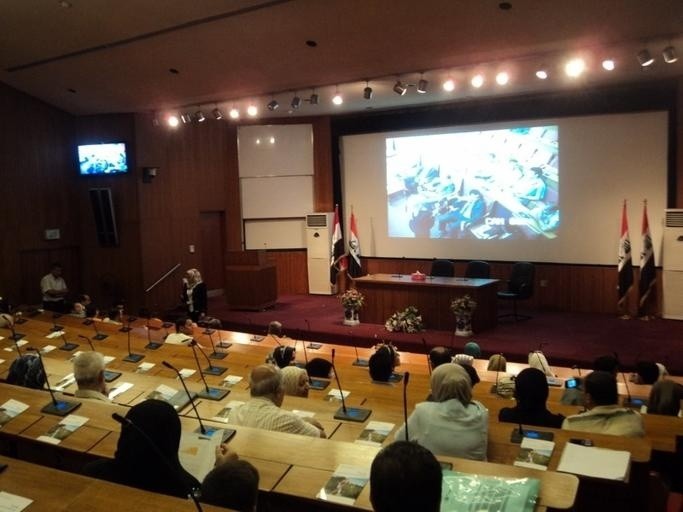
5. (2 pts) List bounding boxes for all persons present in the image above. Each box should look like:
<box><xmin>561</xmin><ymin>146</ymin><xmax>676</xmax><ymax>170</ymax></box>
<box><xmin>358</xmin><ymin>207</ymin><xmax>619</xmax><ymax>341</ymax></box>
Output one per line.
<box><xmin>38</xmin><ymin>263</ymin><xmax>71</xmax><ymax>314</ymax></box>
<box><xmin>181</xmin><ymin>268</ymin><xmax>212</xmax><ymax>318</ymax></box>
<box><xmin>400</xmin><ymin>146</ymin><xmax>558</xmax><ymax>239</ymax></box>
<box><xmin>1</xmin><ymin>294</ymin><xmax>682</xmax><ymax>512</ymax></box>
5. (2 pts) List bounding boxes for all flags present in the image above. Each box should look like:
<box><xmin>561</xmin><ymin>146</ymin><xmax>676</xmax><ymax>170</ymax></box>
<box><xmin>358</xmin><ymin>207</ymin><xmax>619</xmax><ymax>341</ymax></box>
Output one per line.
<box><xmin>639</xmin><ymin>199</ymin><xmax>661</xmax><ymax>312</ymax></box>
<box><xmin>346</xmin><ymin>210</ymin><xmax>362</xmax><ymax>282</ymax></box>
<box><xmin>614</xmin><ymin>200</ymin><xmax>635</xmax><ymax>306</ymax></box>
<box><xmin>328</xmin><ymin>208</ymin><xmax>346</xmax><ymax>282</ymax></box>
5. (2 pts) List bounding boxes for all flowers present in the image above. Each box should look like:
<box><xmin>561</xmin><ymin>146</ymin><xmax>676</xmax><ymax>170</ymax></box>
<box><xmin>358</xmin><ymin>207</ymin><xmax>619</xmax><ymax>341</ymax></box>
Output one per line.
<box><xmin>339</xmin><ymin>287</ymin><xmax>365</xmax><ymax>309</ymax></box>
<box><xmin>445</xmin><ymin>296</ymin><xmax>478</xmax><ymax>315</ymax></box>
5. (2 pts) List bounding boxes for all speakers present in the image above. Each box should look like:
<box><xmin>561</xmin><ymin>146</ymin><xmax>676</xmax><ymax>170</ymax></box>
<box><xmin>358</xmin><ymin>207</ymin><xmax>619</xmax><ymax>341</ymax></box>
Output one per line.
<box><xmin>89</xmin><ymin>188</ymin><xmax>119</xmax><ymax>246</ymax></box>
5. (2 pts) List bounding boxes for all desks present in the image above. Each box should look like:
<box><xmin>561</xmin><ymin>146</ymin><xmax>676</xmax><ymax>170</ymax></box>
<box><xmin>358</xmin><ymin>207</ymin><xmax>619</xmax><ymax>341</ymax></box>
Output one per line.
<box><xmin>352</xmin><ymin>272</ymin><xmax>502</xmax><ymax>330</ymax></box>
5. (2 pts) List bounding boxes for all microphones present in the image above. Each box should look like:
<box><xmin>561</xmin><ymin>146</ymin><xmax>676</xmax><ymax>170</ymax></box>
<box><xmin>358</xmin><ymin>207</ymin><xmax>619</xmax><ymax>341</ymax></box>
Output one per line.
<box><xmin>183</xmin><ymin>277</ymin><xmax>190</xmax><ymax>289</ymax></box>
<box><xmin>426</xmin><ymin>257</ymin><xmax>436</xmax><ymax>279</ymax></box>
<box><xmin>392</xmin><ymin>256</ymin><xmax>406</xmax><ymax>278</ymax></box>
<box><xmin>455</xmin><ymin>262</ymin><xmax>471</xmax><ymax>281</ymax></box>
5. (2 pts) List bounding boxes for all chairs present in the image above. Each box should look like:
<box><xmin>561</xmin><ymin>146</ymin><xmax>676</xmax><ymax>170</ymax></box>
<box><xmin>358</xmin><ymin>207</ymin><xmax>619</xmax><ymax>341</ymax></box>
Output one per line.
<box><xmin>429</xmin><ymin>261</ymin><xmax>457</xmax><ymax>277</ymax></box>
<box><xmin>497</xmin><ymin>262</ymin><xmax>535</xmax><ymax>321</ymax></box>
<box><xmin>465</xmin><ymin>261</ymin><xmax>492</xmax><ymax>281</ymax></box>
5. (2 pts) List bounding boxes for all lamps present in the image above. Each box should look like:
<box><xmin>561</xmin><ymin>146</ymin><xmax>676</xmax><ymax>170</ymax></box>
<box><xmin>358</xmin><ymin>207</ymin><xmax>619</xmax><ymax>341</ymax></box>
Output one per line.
<box><xmin>149</xmin><ymin>33</ymin><xmax>679</xmax><ymax>128</ymax></box>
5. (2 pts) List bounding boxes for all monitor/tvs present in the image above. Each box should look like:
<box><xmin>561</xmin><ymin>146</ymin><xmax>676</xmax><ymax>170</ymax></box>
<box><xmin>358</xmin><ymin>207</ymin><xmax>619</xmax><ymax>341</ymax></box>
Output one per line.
<box><xmin>76</xmin><ymin>142</ymin><xmax>129</xmax><ymax>176</ymax></box>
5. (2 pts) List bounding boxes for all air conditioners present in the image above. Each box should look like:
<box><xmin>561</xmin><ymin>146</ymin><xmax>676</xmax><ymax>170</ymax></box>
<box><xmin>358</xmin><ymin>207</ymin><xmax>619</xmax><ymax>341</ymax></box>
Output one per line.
<box><xmin>306</xmin><ymin>213</ymin><xmax>338</xmax><ymax>296</ymax></box>
<box><xmin>660</xmin><ymin>209</ymin><xmax>682</xmax><ymax>321</ymax></box>
